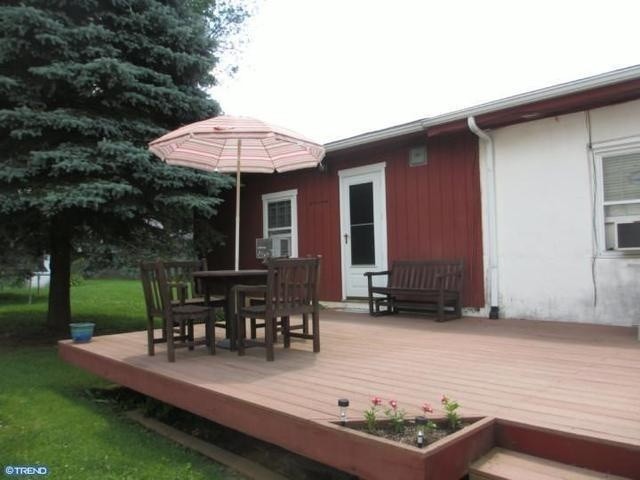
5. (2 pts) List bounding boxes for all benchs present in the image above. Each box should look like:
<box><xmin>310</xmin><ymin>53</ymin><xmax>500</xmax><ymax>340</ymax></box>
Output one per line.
<box><xmin>363</xmin><ymin>258</ymin><xmax>466</xmax><ymax>323</ymax></box>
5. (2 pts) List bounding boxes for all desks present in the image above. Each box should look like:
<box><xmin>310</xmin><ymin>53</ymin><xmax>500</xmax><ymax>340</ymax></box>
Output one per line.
<box><xmin>189</xmin><ymin>269</ymin><xmax>280</xmax><ymax>354</ymax></box>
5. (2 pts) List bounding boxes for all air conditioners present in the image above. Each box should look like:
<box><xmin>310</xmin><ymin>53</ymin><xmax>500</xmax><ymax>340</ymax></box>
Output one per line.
<box><xmin>255</xmin><ymin>238</ymin><xmax>291</xmax><ymax>259</ymax></box>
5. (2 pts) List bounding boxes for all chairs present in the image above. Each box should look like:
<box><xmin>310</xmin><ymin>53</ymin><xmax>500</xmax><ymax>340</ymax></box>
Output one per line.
<box><xmin>238</xmin><ymin>254</ymin><xmax>321</xmax><ymax>361</ymax></box>
<box><xmin>140</xmin><ymin>257</ymin><xmax>226</xmax><ymax>363</ymax></box>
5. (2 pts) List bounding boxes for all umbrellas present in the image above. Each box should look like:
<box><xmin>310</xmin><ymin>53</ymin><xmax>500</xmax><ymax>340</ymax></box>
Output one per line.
<box><xmin>148</xmin><ymin>117</ymin><xmax>326</xmax><ymax>271</ymax></box>
